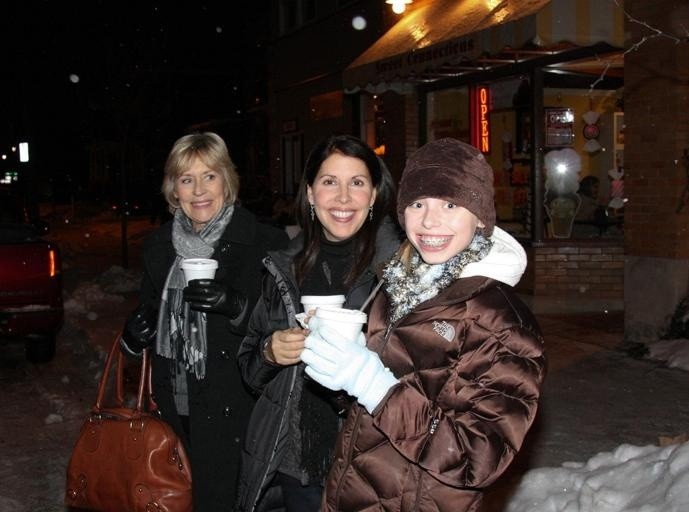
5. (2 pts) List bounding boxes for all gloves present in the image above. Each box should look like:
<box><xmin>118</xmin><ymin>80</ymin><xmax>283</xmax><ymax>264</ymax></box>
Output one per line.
<box><xmin>120</xmin><ymin>303</ymin><xmax>159</xmax><ymax>352</ymax></box>
<box><xmin>182</xmin><ymin>278</ymin><xmax>248</xmax><ymax>319</ymax></box>
<box><xmin>301</xmin><ymin>327</ymin><xmax>401</xmax><ymax>415</ymax></box>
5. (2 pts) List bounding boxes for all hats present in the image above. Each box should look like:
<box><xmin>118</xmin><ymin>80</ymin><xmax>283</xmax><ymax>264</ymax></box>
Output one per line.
<box><xmin>394</xmin><ymin>136</ymin><xmax>498</xmax><ymax>239</ymax></box>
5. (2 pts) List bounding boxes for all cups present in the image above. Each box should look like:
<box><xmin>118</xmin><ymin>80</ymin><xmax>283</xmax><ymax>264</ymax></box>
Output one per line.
<box><xmin>314</xmin><ymin>305</ymin><xmax>369</xmax><ymax>348</ymax></box>
<box><xmin>180</xmin><ymin>259</ymin><xmax>219</xmax><ymax>285</ymax></box>
<box><xmin>300</xmin><ymin>294</ymin><xmax>345</xmax><ymax>318</ymax></box>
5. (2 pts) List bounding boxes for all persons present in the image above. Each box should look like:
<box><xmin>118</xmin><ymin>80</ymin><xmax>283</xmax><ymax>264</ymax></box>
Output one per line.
<box><xmin>117</xmin><ymin>131</ymin><xmax>278</xmax><ymax>511</ymax></box>
<box><xmin>573</xmin><ymin>175</ymin><xmax>616</xmax><ymax>219</ymax></box>
<box><xmin>235</xmin><ymin>135</ymin><xmax>405</xmax><ymax>511</ymax></box>
<box><xmin>299</xmin><ymin>137</ymin><xmax>548</xmax><ymax>511</ymax></box>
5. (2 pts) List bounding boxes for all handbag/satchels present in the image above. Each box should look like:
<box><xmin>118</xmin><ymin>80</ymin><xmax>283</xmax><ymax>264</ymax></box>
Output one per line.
<box><xmin>62</xmin><ymin>404</ymin><xmax>194</xmax><ymax>512</ymax></box>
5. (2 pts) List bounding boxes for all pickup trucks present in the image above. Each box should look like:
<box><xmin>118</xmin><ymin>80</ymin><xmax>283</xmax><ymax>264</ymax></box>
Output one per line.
<box><xmin>2</xmin><ymin>187</ymin><xmax>64</xmax><ymax>363</ymax></box>
